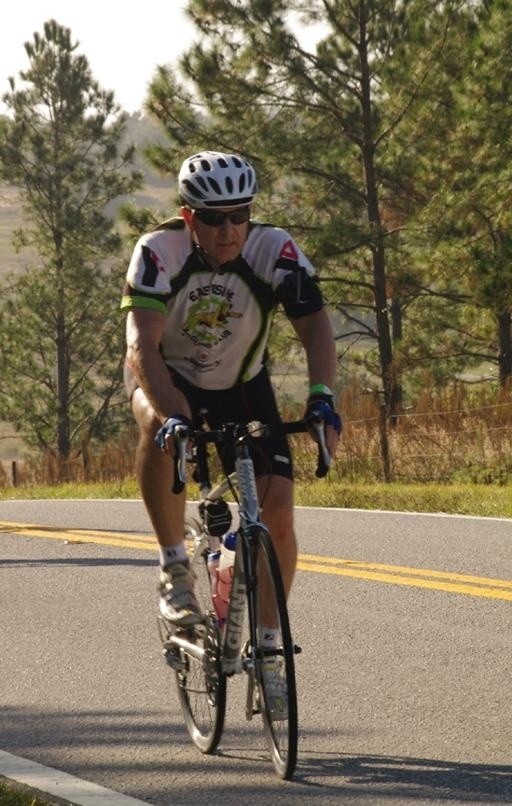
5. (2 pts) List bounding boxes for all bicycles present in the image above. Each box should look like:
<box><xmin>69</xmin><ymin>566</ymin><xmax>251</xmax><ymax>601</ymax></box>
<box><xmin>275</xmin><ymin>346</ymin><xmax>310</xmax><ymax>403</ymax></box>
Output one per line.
<box><xmin>157</xmin><ymin>409</ymin><xmax>332</xmax><ymax>783</ymax></box>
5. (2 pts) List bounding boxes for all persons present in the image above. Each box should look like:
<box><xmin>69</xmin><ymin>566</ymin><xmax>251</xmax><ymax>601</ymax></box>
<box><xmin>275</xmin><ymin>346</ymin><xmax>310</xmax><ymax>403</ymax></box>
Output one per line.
<box><xmin>120</xmin><ymin>151</ymin><xmax>342</xmax><ymax>721</ymax></box>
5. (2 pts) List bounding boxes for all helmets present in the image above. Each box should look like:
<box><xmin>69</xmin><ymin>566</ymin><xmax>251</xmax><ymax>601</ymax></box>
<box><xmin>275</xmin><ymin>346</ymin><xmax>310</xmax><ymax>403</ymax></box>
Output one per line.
<box><xmin>177</xmin><ymin>151</ymin><xmax>258</xmax><ymax>210</ymax></box>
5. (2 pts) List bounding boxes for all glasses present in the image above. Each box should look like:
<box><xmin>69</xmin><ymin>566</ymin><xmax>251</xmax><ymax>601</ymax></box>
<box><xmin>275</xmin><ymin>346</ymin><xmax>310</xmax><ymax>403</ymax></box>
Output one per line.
<box><xmin>194</xmin><ymin>208</ymin><xmax>250</xmax><ymax>226</ymax></box>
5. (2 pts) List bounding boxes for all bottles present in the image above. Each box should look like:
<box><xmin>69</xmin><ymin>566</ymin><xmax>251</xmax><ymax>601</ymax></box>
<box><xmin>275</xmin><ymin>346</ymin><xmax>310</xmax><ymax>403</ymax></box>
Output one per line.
<box><xmin>205</xmin><ymin>550</ymin><xmax>229</xmax><ymax>620</ymax></box>
<box><xmin>218</xmin><ymin>529</ymin><xmax>239</xmax><ymax>603</ymax></box>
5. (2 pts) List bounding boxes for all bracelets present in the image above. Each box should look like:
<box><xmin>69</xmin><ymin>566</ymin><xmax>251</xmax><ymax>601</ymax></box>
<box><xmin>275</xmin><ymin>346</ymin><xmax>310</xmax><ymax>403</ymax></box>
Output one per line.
<box><xmin>310</xmin><ymin>384</ymin><xmax>333</xmax><ymax>396</ymax></box>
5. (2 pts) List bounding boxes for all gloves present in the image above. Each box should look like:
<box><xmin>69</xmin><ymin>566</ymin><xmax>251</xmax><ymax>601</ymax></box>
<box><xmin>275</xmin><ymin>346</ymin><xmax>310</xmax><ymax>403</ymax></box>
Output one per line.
<box><xmin>303</xmin><ymin>394</ymin><xmax>341</xmax><ymax>436</ymax></box>
<box><xmin>154</xmin><ymin>413</ymin><xmax>196</xmax><ymax>448</ymax></box>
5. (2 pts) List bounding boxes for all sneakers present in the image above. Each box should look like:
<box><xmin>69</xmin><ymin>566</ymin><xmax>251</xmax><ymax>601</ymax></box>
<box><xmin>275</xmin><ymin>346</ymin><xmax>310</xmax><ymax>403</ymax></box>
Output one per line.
<box><xmin>158</xmin><ymin>562</ymin><xmax>203</xmax><ymax>626</ymax></box>
<box><xmin>262</xmin><ymin>659</ymin><xmax>288</xmax><ymax>721</ymax></box>
